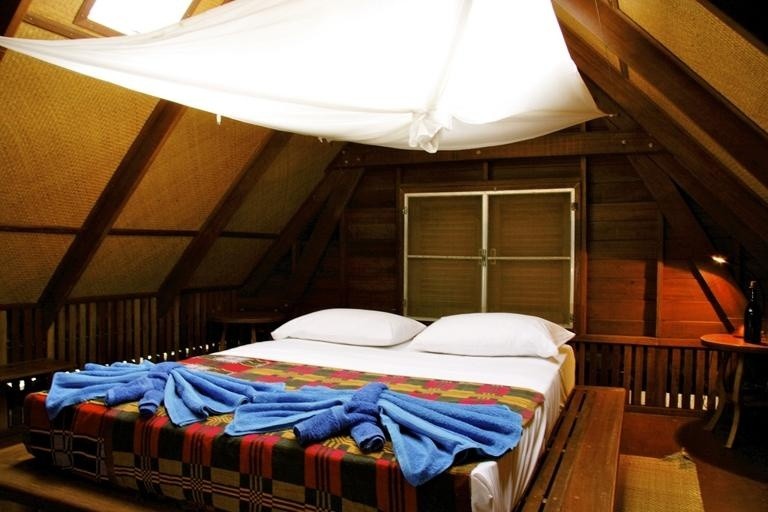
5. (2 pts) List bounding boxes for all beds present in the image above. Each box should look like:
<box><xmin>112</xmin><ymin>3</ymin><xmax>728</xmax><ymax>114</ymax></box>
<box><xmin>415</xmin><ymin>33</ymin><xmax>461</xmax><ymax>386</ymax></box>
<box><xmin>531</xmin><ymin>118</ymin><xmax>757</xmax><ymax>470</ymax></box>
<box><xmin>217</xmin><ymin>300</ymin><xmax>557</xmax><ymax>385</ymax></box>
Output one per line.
<box><xmin>20</xmin><ymin>342</ymin><xmax>578</xmax><ymax>512</ymax></box>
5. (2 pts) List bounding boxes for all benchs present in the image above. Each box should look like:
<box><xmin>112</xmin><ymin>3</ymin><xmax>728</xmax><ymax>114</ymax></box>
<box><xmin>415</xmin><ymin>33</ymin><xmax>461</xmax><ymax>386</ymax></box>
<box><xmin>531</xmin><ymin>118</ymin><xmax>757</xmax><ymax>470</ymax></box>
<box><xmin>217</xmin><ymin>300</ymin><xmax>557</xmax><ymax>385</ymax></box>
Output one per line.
<box><xmin>510</xmin><ymin>386</ymin><xmax>626</xmax><ymax>511</ymax></box>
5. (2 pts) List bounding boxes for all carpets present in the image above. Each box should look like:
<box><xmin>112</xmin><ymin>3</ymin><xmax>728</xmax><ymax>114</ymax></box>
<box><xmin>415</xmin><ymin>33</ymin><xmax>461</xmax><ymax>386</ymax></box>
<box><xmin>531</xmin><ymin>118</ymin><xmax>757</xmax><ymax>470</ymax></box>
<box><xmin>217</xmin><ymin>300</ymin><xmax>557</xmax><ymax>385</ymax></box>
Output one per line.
<box><xmin>616</xmin><ymin>447</ymin><xmax>705</xmax><ymax>512</ymax></box>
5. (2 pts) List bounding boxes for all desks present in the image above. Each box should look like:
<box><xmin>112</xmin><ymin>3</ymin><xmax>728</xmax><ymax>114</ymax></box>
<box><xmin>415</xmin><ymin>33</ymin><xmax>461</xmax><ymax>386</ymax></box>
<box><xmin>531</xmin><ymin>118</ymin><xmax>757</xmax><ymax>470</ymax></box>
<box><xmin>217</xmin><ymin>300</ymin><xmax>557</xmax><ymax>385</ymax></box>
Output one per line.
<box><xmin>698</xmin><ymin>331</ymin><xmax>768</xmax><ymax>450</ymax></box>
<box><xmin>206</xmin><ymin>310</ymin><xmax>285</xmax><ymax>352</ymax></box>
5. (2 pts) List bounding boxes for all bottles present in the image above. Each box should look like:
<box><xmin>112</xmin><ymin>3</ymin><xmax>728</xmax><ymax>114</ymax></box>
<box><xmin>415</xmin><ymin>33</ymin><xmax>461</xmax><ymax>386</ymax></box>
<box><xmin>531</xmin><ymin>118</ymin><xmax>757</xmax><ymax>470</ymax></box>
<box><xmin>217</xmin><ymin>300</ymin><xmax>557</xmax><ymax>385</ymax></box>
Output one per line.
<box><xmin>743</xmin><ymin>281</ymin><xmax>763</xmax><ymax>344</ymax></box>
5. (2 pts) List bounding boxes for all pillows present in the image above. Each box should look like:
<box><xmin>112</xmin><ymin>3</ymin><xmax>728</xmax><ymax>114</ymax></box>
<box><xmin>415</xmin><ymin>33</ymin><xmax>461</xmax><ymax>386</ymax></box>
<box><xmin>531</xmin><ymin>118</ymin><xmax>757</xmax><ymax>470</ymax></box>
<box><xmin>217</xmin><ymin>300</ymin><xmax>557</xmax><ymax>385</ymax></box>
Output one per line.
<box><xmin>271</xmin><ymin>308</ymin><xmax>427</xmax><ymax>347</ymax></box>
<box><xmin>405</xmin><ymin>313</ymin><xmax>577</xmax><ymax>359</ymax></box>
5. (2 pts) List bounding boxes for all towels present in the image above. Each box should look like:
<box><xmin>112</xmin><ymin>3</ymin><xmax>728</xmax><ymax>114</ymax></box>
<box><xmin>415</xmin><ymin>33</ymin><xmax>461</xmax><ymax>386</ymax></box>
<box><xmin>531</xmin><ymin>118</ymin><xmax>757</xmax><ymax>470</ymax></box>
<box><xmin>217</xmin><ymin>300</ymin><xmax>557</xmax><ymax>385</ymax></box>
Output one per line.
<box><xmin>226</xmin><ymin>360</ymin><xmax>525</xmax><ymax>485</ymax></box>
<box><xmin>45</xmin><ymin>359</ymin><xmax>286</xmax><ymax>436</ymax></box>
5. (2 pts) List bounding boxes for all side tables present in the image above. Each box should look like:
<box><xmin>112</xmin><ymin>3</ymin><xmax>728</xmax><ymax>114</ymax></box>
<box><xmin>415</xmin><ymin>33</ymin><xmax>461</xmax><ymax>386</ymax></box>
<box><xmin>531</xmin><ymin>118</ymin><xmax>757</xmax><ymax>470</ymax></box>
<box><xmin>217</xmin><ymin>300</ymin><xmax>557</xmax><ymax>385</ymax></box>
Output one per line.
<box><xmin>0</xmin><ymin>358</ymin><xmax>76</xmax><ymax>444</ymax></box>
